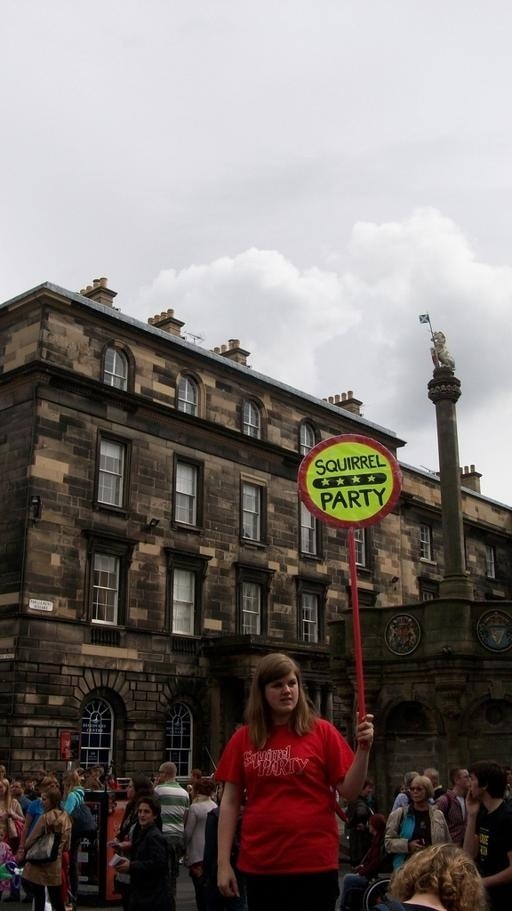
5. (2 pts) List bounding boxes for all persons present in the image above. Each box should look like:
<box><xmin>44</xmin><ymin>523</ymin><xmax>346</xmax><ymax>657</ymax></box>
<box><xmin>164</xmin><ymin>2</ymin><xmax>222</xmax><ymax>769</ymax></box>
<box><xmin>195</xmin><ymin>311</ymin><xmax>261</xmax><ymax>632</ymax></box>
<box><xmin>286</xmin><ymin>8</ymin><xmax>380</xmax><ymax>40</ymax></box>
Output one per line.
<box><xmin>214</xmin><ymin>649</ymin><xmax>377</xmax><ymax>910</ymax></box>
<box><xmin>430</xmin><ymin>342</ymin><xmax>444</xmax><ymax>369</ymax></box>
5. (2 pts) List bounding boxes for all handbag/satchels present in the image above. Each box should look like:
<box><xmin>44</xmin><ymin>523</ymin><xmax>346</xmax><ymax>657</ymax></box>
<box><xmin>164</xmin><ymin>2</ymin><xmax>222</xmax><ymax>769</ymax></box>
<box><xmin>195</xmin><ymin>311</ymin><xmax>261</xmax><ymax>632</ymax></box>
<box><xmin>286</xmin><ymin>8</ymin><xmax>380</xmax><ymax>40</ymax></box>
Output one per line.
<box><xmin>21</xmin><ymin>812</ymin><xmax>61</xmax><ymax>865</ymax></box>
<box><xmin>68</xmin><ymin>798</ymin><xmax>100</xmax><ymax>837</ymax></box>
<box><xmin>7</xmin><ymin>810</ymin><xmax>24</xmax><ymax>840</ymax></box>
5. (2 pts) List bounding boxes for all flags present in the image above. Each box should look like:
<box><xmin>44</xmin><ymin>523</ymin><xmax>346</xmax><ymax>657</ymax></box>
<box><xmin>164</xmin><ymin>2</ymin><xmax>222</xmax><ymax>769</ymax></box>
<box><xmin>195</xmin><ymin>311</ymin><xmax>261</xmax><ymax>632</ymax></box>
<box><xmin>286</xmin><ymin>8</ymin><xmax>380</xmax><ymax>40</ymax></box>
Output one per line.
<box><xmin>418</xmin><ymin>314</ymin><xmax>430</xmax><ymax>325</ymax></box>
